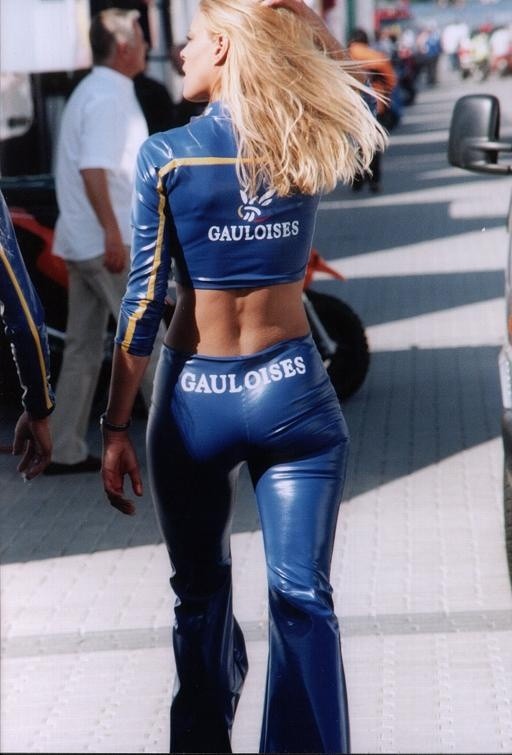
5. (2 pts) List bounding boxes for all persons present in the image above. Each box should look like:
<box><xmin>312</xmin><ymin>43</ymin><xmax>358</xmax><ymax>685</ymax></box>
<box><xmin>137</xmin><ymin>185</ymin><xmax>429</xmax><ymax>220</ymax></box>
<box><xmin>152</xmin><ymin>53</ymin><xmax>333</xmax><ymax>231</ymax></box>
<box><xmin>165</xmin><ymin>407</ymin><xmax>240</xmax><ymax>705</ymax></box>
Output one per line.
<box><xmin>0</xmin><ymin>181</ymin><xmax>57</xmax><ymax>480</ymax></box>
<box><xmin>47</xmin><ymin>4</ymin><xmax>153</xmax><ymax>472</ymax></box>
<box><xmin>338</xmin><ymin>19</ymin><xmax>512</xmax><ymax>193</ymax></box>
<box><xmin>97</xmin><ymin>0</ymin><xmax>393</xmax><ymax>752</ymax></box>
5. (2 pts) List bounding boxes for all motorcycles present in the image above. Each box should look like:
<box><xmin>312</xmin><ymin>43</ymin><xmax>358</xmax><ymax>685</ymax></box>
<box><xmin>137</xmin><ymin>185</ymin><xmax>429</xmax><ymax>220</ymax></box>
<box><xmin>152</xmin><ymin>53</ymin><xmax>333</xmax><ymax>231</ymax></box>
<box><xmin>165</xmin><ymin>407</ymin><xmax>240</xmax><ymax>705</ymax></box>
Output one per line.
<box><xmin>0</xmin><ymin>200</ymin><xmax>370</xmax><ymax>418</ymax></box>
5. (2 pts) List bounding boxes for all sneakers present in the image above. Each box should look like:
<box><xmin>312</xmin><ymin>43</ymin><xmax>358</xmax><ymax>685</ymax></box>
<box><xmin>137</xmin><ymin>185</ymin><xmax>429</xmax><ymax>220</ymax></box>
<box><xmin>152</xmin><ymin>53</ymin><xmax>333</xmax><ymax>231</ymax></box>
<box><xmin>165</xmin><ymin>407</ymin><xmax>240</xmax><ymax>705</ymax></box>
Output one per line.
<box><xmin>41</xmin><ymin>454</ymin><xmax>103</xmax><ymax>477</ymax></box>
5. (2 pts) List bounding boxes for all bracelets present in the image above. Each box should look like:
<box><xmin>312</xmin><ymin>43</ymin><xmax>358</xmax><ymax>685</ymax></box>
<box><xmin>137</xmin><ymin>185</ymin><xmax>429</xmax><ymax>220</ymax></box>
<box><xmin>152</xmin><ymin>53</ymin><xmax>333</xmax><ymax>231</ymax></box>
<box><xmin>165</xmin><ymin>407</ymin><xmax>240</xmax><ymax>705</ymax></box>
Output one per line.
<box><xmin>100</xmin><ymin>412</ymin><xmax>132</xmax><ymax>432</ymax></box>
<box><xmin>323</xmin><ymin>38</ymin><xmax>337</xmax><ymax>51</ymax></box>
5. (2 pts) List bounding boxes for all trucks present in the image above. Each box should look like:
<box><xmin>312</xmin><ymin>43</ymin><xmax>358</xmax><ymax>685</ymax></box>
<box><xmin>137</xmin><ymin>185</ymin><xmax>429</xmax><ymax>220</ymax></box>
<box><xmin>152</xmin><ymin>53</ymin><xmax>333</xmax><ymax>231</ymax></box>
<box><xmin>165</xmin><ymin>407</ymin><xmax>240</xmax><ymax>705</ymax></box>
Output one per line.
<box><xmin>445</xmin><ymin>94</ymin><xmax>511</xmax><ymax>591</ymax></box>
